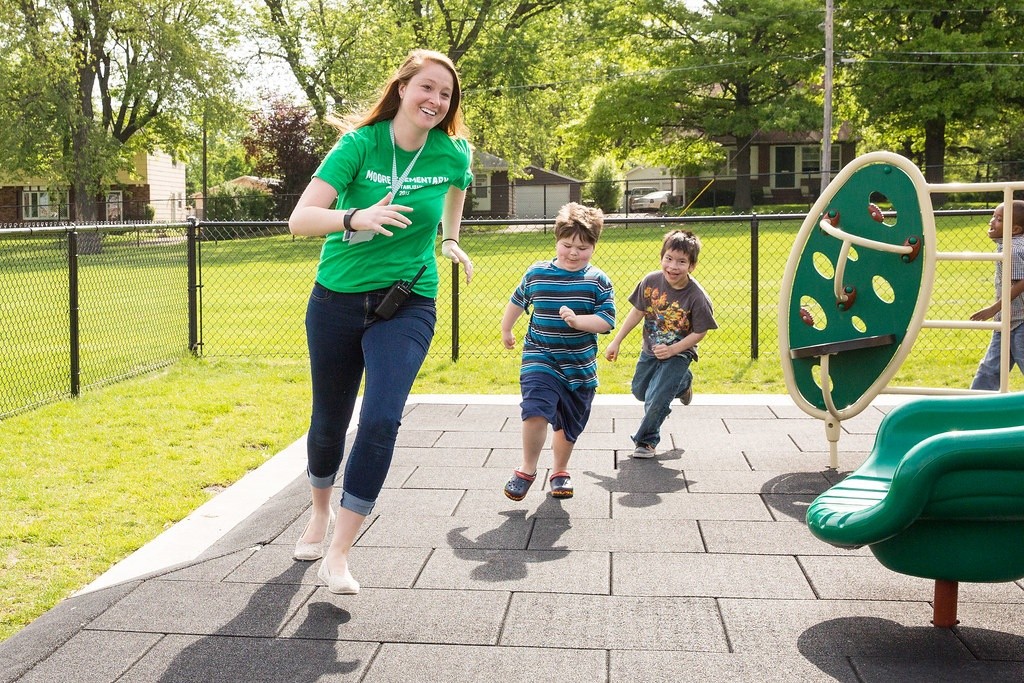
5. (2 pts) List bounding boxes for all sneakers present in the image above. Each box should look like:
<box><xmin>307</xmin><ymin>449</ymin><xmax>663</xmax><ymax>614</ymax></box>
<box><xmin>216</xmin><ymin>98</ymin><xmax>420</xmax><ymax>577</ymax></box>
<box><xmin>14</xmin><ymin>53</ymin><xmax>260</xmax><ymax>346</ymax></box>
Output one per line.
<box><xmin>633</xmin><ymin>441</ymin><xmax>655</xmax><ymax>458</ymax></box>
<box><xmin>680</xmin><ymin>370</ymin><xmax>692</xmax><ymax>404</ymax></box>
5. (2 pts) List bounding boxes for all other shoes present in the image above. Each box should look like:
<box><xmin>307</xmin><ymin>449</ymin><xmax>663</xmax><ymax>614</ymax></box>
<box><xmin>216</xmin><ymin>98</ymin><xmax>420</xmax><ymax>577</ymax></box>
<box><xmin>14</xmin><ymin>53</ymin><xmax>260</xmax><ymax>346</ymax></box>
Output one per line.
<box><xmin>318</xmin><ymin>556</ymin><xmax>360</xmax><ymax>593</ymax></box>
<box><xmin>294</xmin><ymin>504</ymin><xmax>335</xmax><ymax>560</ymax></box>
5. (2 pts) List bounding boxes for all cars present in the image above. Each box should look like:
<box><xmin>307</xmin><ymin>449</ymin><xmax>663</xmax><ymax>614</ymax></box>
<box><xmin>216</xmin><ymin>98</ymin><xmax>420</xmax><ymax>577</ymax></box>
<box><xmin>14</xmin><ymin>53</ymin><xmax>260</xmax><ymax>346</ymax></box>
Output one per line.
<box><xmin>631</xmin><ymin>190</ymin><xmax>672</xmax><ymax>212</ymax></box>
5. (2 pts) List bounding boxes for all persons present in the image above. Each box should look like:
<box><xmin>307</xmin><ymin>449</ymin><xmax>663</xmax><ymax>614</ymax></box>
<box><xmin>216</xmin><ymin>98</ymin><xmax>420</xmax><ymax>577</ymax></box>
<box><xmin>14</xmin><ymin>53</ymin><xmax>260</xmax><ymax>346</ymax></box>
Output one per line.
<box><xmin>289</xmin><ymin>49</ymin><xmax>474</xmax><ymax>594</ymax></box>
<box><xmin>502</xmin><ymin>202</ymin><xmax>616</xmax><ymax>501</ymax></box>
<box><xmin>605</xmin><ymin>229</ymin><xmax>718</xmax><ymax>458</ymax></box>
<box><xmin>970</xmin><ymin>200</ymin><xmax>1024</xmax><ymax>390</ymax></box>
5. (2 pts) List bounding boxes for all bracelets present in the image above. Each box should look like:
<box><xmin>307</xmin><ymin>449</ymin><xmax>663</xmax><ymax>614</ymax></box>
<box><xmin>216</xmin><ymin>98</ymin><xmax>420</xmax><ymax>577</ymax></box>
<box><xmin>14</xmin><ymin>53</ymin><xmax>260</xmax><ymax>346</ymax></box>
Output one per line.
<box><xmin>344</xmin><ymin>207</ymin><xmax>360</xmax><ymax>232</ymax></box>
<box><xmin>442</xmin><ymin>238</ymin><xmax>459</xmax><ymax>246</ymax></box>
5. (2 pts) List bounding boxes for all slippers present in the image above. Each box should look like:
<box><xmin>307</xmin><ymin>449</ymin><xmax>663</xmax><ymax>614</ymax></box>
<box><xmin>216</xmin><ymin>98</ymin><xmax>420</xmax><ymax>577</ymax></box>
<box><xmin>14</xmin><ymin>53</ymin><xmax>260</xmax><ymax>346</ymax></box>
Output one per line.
<box><xmin>550</xmin><ymin>471</ymin><xmax>573</xmax><ymax>498</ymax></box>
<box><xmin>504</xmin><ymin>466</ymin><xmax>538</xmax><ymax>501</ymax></box>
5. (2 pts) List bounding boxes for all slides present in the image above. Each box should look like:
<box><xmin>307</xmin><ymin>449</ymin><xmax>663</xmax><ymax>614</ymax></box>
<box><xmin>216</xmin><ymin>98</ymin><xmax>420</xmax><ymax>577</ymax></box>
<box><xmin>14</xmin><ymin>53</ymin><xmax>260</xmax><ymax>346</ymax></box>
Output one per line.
<box><xmin>805</xmin><ymin>384</ymin><xmax>1024</xmax><ymax>550</ymax></box>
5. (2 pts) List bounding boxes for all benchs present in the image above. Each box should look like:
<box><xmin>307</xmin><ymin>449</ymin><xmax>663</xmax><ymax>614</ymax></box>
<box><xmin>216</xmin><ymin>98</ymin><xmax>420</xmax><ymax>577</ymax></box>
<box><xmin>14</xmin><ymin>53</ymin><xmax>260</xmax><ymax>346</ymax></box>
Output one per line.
<box><xmin>770</xmin><ymin>187</ymin><xmax>815</xmax><ymax>210</ymax></box>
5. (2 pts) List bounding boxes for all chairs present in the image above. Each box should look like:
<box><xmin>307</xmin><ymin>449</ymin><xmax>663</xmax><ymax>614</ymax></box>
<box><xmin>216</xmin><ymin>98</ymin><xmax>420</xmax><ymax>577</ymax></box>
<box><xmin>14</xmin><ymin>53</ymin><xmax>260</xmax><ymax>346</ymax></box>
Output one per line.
<box><xmin>804</xmin><ymin>391</ymin><xmax>1024</xmax><ymax>627</ymax></box>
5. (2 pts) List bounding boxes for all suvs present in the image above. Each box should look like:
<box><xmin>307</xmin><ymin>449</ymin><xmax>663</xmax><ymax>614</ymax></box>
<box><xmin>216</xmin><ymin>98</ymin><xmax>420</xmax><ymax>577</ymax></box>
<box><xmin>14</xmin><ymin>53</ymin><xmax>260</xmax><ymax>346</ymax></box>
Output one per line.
<box><xmin>629</xmin><ymin>187</ymin><xmax>659</xmax><ymax>211</ymax></box>
